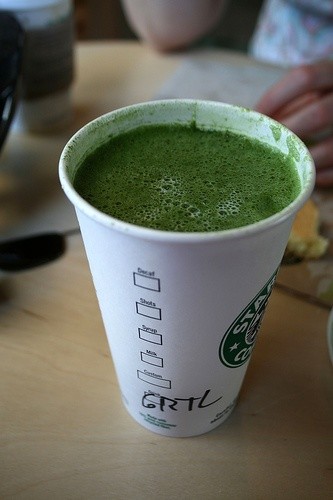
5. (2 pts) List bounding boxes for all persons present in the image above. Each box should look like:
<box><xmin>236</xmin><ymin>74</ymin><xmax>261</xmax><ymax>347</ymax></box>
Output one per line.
<box><xmin>122</xmin><ymin>0</ymin><xmax>332</xmax><ymax>192</ymax></box>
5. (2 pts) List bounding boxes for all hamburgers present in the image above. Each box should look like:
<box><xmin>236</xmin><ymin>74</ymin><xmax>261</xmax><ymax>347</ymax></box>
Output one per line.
<box><xmin>281</xmin><ymin>200</ymin><xmax>329</xmax><ymax>265</ymax></box>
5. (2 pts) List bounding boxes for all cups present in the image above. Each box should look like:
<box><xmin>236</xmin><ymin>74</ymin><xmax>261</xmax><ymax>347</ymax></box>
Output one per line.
<box><xmin>58</xmin><ymin>98</ymin><xmax>315</xmax><ymax>438</ymax></box>
<box><xmin>1</xmin><ymin>0</ymin><xmax>77</xmax><ymax>132</ymax></box>
